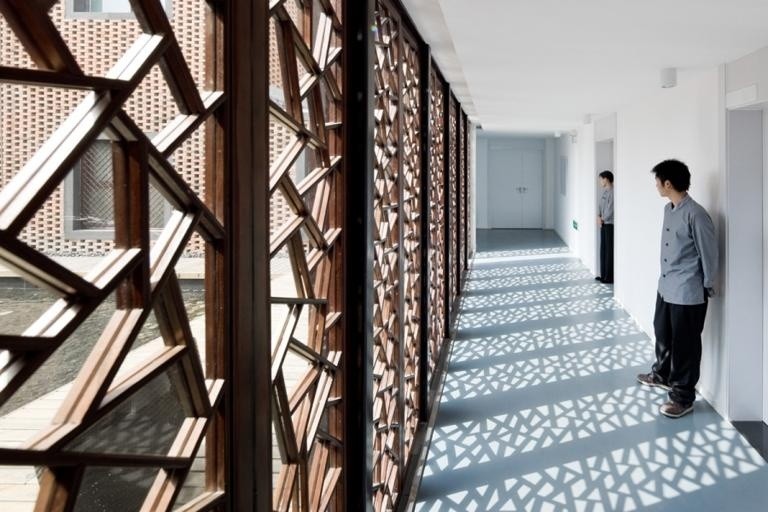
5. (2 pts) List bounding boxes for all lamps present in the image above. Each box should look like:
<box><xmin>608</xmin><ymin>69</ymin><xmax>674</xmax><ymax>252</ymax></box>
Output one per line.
<box><xmin>661</xmin><ymin>63</ymin><xmax>679</xmax><ymax>90</ymax></box>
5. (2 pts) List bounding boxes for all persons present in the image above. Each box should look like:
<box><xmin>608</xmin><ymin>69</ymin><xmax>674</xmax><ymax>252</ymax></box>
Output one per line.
<box><xmin>595</xmin><ymin>170</ymin><xmax>614</xmax><ymax>283</ymax></box>
<box><xmin>635</xmin><ymin>159</ymin><xmax>719</xmax><ymax>418</ymax></box>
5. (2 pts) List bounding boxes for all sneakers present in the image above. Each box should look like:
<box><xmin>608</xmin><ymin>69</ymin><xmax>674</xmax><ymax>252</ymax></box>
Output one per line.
<box><xmin>595</xmin><ymin>277</ymin><xmax>609</xmax><ymax>283</ymax></box>
<box><xmin>637</xmin><ymin>373</ymin><xmax>672</xmax><ymax>391</ymax></box>
<box><xmin>660</xmin><ymin>399</ymin><xmax>694</xmax><ymax>418</ymax></box>
